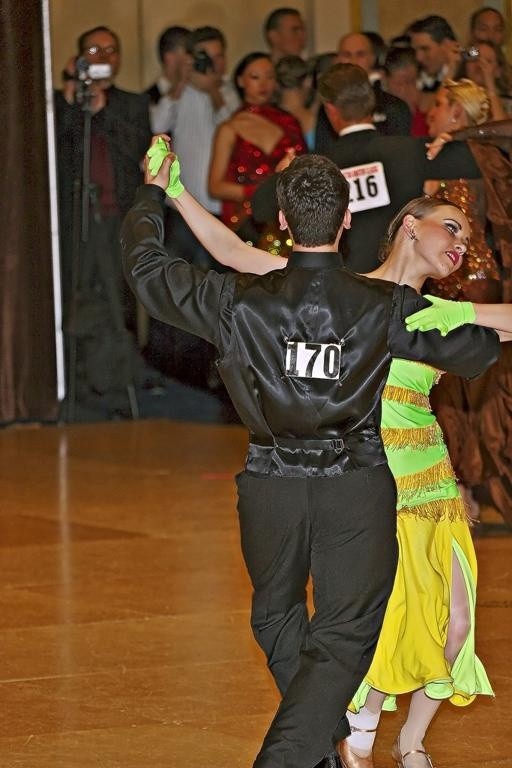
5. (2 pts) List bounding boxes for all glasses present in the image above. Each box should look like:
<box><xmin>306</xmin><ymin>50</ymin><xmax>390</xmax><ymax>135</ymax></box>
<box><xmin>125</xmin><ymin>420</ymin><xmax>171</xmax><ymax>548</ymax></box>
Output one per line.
<box><xmin>84</xmin><ymin>45</ymin><xmax>117</xmax><ymax>55</ymax></box>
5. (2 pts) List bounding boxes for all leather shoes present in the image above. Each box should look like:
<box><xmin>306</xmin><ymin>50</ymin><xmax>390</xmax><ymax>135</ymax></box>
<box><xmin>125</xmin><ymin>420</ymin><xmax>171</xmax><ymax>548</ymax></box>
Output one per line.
<box><xmin>315</xmin><ymin>755</ymin><xmax>341</xmax><ymax>768</ymax></box>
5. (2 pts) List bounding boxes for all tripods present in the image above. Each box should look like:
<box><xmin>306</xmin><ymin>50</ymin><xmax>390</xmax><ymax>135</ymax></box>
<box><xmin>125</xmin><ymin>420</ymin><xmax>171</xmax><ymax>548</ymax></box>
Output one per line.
<box><xmin>65</xmin><ymin>86</ymin><xmax>140</xmax><ymax>426</ymax></box>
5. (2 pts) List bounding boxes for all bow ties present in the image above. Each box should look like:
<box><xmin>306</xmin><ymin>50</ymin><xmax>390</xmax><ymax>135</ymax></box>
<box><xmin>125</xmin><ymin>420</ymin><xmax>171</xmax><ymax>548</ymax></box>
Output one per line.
<box><xmin>420</xmin><ymin>64</ymin><xmax>450</xmax><ymax>89</ymax></box>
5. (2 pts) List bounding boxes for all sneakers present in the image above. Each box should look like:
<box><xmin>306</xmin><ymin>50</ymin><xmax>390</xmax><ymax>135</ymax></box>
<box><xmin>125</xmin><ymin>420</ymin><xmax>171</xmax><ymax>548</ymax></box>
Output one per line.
<box><xmin>140</xmin><ymin>372</ymin><xmax>167</xmax><ymax>398</ymax></box>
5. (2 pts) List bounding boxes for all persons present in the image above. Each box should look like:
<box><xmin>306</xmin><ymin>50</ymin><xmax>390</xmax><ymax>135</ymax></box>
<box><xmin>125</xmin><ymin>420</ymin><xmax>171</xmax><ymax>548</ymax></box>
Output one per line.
<box><xmin>426</xmin><ymin>78</ymin><xmax>511</xmax><ymax>531</ymax></box>
<box><xmin>59</xmin><ymin>8</ymin><xmax>511</xmax><ymax>423</ymax></box>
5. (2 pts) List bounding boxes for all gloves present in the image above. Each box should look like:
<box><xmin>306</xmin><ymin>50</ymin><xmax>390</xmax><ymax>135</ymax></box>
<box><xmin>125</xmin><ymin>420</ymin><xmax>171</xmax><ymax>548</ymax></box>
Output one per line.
<box><xmin>424</xmin><ymin>132</ymin><xmax>453</xmax><ymax>161</ymax></box>
<box><xmin>405</xmin><ymin>294</ymin><xmax>476</xmax><ymax>338</ymax></box>
<box><xmin>149</xmin><ymin>140</ymin><xmax>184</xmax><ymax>199</ymax></box>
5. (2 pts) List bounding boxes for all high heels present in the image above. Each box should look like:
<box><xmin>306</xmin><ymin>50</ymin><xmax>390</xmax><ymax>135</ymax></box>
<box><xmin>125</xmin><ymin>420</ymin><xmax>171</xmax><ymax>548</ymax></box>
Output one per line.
<box><xmin>392</xmin><ymin>735</ymin><xmax>436</xmax><ymax>768</ymax></box>
<box><xmin>335</xmin><ymin>726</ymin><xmax>378</xmax><ymax>768</ymax></box>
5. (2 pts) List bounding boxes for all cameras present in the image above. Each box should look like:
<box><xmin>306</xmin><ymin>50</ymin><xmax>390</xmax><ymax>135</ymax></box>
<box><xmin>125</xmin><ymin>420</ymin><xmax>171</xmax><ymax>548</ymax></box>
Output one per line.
<box><xmin>193</xmin><ymin>50</ymin><xmax>212</xmax><ymax>74</ymax></box>
<box><xmin>461</xmin><ymin>47</ymin><xmax>480</xmax><ymax>57</ymax></box>
<box><xmin>74</xmin><ymin>56</ymin><xmax>112</xmax><ymax>86</ymax></box>
